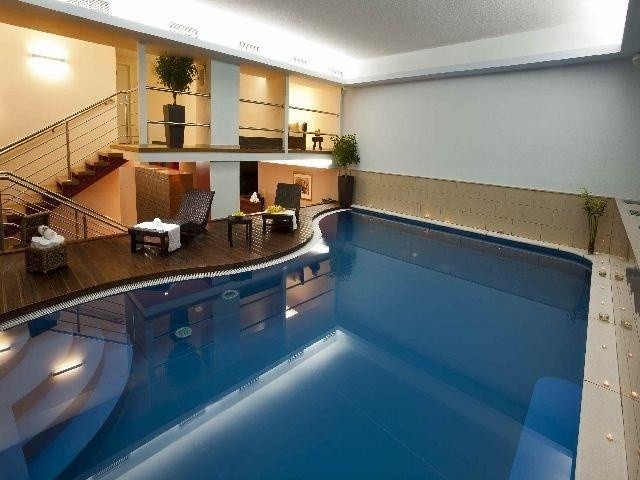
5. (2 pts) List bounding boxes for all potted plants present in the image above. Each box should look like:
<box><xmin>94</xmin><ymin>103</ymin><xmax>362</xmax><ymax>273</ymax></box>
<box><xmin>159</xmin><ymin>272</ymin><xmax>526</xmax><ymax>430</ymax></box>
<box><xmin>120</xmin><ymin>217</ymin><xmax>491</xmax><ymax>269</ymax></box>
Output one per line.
<box><xmin>331</xmin><ymin>132</ymin><xmax>361</xmax><ymax>208</ymax></box>
<box><xmin>579</xmin><ymin>187</ymin><xmax>610</xmax><ymax>256</ymax></box>
<box><xmin>155</xmin><ymin>53</ymin><xmax>199</xmax><ymax>147</ymax></box>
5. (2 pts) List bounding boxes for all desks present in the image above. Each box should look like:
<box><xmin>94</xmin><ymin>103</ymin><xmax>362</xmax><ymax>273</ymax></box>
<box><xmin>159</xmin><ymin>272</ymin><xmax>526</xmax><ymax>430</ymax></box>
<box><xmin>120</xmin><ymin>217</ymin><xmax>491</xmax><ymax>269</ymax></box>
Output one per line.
<box><xmin>228</xmin><ymin>213</ymin><xmax>253</xmax><ymax>248</ymax></box>
<box><xmin>312</xmin><ymin>136</ymin><xmax>323</xmax><ymax>151</ymax></box>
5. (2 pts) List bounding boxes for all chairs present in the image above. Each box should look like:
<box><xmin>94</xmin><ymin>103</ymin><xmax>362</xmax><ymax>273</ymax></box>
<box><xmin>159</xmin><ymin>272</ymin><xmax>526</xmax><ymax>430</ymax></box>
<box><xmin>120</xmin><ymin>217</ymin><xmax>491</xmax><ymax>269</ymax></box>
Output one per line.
<box><xmin>263</xmin><ymin>183</ymin><xmax>303</xmax><ymax>236</ymax></box>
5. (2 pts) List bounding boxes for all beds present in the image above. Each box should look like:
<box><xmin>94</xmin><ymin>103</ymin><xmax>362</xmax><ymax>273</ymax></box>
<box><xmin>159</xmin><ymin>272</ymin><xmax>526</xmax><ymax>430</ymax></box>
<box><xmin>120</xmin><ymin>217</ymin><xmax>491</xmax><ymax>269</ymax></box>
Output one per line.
<box><xmin>127</xmin><ymin>190</ymin><xmax>215</xmax><ymax>254</ymax></box>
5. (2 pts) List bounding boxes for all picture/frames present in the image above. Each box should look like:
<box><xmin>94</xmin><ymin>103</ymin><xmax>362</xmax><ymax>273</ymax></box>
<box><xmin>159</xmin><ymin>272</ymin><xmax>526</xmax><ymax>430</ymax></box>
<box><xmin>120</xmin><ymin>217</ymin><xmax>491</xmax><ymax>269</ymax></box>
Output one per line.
<box><xmin>293</xmin><ymin>171</ymin><xmax>313</xmax><ymax>201</ymax></box>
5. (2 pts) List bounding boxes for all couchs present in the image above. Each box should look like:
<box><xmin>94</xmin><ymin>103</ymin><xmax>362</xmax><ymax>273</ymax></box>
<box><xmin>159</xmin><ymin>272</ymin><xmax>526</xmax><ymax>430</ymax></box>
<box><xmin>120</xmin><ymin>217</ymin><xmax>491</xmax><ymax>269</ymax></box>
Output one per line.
<box><xmin>288</xmin><ymin>121</ymin><xmax>307</xmax><ymax>150</ymax></box>
